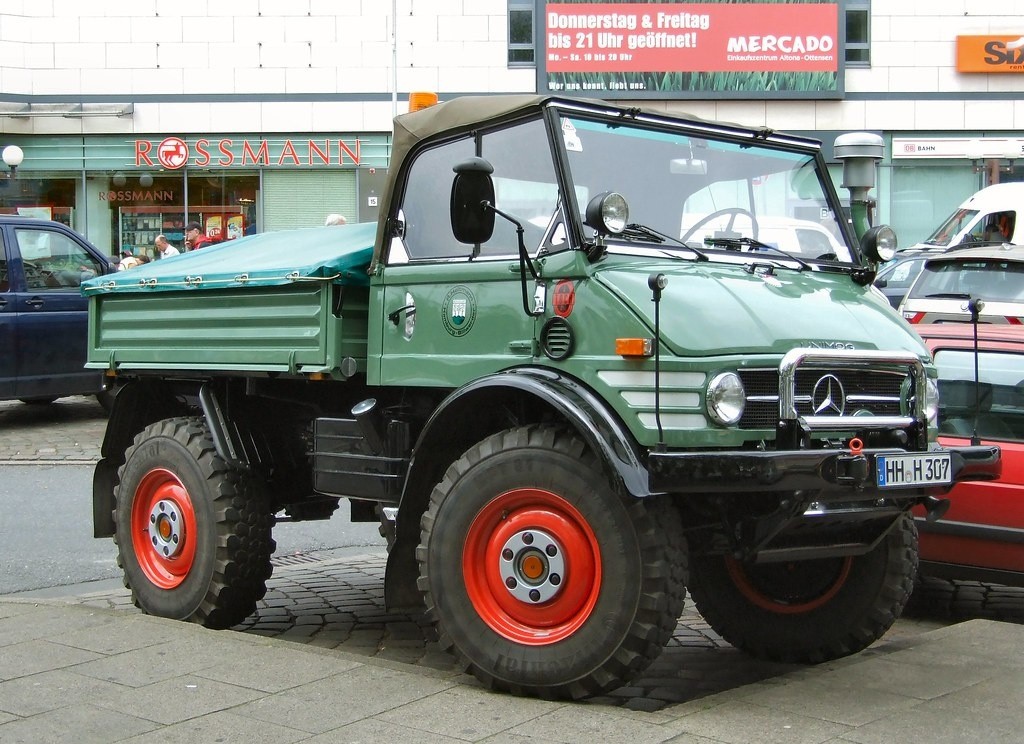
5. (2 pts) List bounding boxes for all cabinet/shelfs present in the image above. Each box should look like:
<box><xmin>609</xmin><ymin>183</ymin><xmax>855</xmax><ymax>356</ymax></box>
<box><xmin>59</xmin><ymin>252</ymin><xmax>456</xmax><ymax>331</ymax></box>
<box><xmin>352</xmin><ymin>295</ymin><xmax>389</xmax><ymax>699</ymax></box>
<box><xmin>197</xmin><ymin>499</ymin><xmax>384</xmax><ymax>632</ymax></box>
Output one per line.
<box><xmin>119</xmin><ymin>207</ymin><xmax>185</xmax><ymax>247</ymax></box>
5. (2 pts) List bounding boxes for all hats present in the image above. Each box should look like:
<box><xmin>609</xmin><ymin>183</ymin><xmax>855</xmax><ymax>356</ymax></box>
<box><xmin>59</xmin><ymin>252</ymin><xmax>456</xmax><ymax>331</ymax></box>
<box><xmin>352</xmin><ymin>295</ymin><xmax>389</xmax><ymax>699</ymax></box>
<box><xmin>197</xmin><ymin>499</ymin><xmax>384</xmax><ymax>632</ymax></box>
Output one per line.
<box><xmin>182</xmin><ymin>221</ymin><xmax>202</xmax><ymax>232</ymax></box>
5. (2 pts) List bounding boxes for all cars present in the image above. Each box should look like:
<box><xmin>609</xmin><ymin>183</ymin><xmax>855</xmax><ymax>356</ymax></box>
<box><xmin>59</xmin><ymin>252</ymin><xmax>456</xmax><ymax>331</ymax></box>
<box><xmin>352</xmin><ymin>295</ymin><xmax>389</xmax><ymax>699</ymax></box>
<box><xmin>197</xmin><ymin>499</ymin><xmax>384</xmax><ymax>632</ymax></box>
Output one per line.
<box><xmin>519</xmin><ymin>173</ymin><xmax>1024</xmax><ymax>618</ymax></box>
<box><xmin>0</xmin><ymin>215</ymin><xmax>129</xmax><ymax>415</ymax></box>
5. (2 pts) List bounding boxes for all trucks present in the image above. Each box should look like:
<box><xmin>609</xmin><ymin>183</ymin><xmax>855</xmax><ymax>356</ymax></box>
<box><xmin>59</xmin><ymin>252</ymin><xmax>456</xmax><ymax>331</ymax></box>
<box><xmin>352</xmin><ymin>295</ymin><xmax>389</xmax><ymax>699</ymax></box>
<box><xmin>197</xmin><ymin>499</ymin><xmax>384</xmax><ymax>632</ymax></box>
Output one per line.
<box><xmin>84</xmin><ymin>90</ymin><xmax>1001</xmax><ymax>699</ymax></box>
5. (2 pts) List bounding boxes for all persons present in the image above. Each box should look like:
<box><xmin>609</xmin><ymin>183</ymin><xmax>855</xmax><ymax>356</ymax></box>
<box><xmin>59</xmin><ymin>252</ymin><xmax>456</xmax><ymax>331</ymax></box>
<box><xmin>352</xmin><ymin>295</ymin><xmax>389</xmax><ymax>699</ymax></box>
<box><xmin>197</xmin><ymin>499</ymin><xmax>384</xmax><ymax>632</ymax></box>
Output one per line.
<box><xmin>983</xmin><ymin>223</ymin><xmax>1008</xmax><ymax>247</ymax></box>
<box><xmin>154</xmin><ymin>235</ymin><xmax>180</xmax><ymax>259</ymax></box>
<box><xmin>325</xmin><ymin>213</ymin><xmax>347</xmax><ymax>227</ymax></box>
<box><xmin>116</xmin><ymin>255</ymin><xmax>149</xmax><ymax>271</ymax></box>
<box><xmin>182</xmin><ymin>221</ymin><xmax>215</xmax><ymax>252</ymax></box>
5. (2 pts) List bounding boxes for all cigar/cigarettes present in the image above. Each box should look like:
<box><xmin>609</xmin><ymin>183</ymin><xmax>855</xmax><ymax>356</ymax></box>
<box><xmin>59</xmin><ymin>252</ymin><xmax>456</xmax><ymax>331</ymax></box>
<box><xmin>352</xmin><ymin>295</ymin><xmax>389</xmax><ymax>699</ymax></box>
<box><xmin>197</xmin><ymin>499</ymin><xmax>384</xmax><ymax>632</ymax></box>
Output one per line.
<box><xmin>183</xmin><ymin>235</ymin><xmax>186</xmax><ymax>238</ymax></box>
